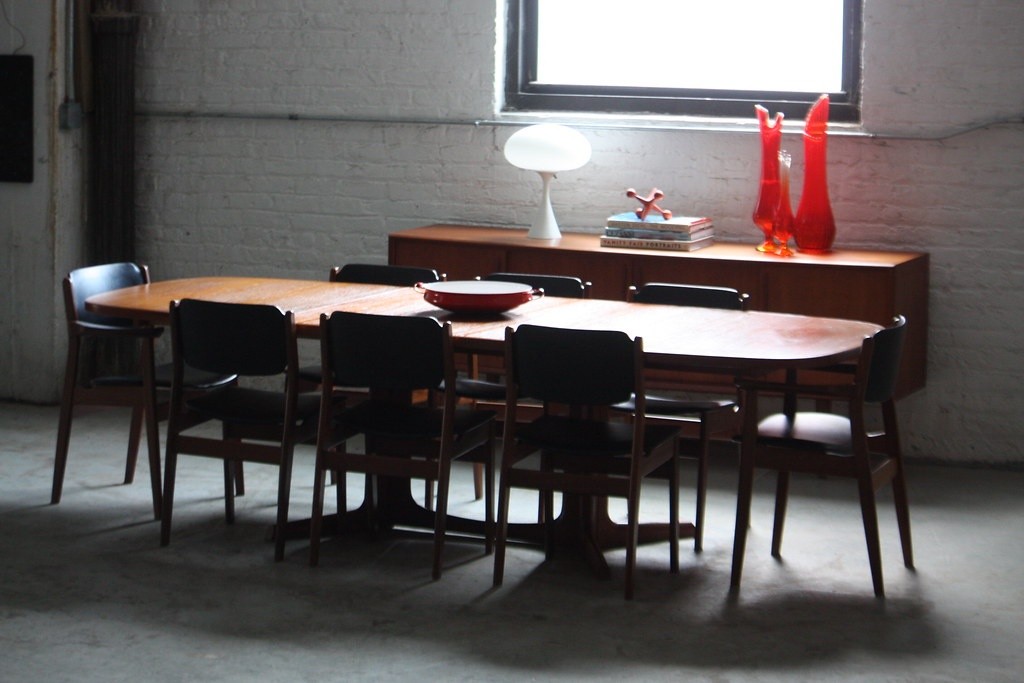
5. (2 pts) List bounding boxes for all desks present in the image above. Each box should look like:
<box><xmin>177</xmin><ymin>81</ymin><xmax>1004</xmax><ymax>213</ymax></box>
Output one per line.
<box><xmin>84</xmin><ymin>277</ymin><xmax>887</xmax><ymax>597</ymax></box>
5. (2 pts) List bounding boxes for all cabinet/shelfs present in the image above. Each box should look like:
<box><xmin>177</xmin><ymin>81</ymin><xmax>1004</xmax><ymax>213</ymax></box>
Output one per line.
<box><xmin>388</xmin><ymin>224</ymin><xmax>930</xmax><ymax>440</ymax></box>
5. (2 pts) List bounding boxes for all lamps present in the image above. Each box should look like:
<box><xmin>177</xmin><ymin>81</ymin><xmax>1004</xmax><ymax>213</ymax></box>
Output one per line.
<box><xmin>504</xmin><ymin>124</ymin><xmax>593</xmax><ymax>241</ymax></box>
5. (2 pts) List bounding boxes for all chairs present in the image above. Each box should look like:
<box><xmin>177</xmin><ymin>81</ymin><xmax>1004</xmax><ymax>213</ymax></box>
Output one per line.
<box><xmin>50</xmin><ymin>263</ymin><xmax>914</xmax><ymax>598</ymax></box>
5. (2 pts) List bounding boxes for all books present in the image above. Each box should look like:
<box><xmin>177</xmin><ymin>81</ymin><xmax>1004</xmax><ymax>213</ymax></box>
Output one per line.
<box><xmin>600</xmin><ymin>210</ymin><xmax>716</xmax><ymax>252</ymax></box>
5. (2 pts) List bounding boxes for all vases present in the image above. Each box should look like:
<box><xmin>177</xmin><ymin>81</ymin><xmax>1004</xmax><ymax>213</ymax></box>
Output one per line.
<box><xmin>752</xmin><ymin>94</ymin><xmax>837</xmax><ymax>257</ymax></box>
